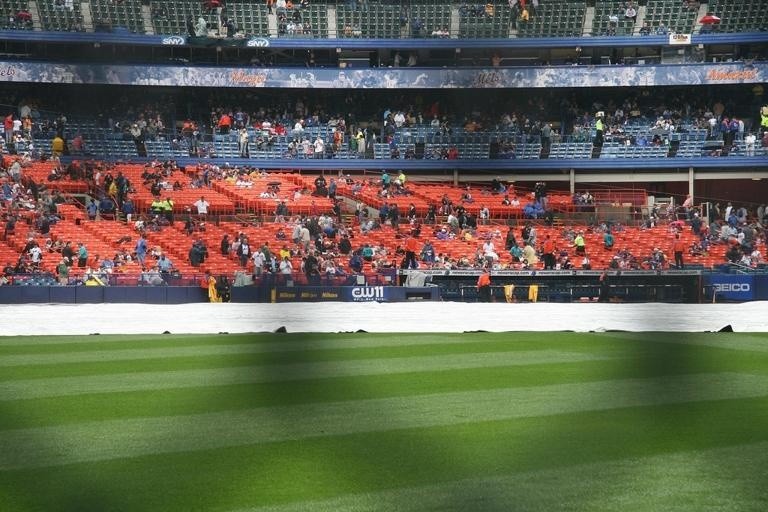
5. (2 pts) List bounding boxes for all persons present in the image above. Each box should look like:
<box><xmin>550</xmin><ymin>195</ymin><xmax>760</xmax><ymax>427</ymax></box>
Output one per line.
<box><xmin>1</xmin><ymin>0</ymin><xmax>768</xmax><ymax>302</ymax></box>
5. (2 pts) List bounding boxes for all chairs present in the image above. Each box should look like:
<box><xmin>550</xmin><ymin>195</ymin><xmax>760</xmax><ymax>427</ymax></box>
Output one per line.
<box><xmin>1</xmin><ymin>103</ymin><xmax>768</xmax><ymax>157</ymax></box>
<box><xmin>0</xmin><ymin>155</ymin><xmax>767</xmax><ymax>285</ymax></box>
<box><xmin>0</xmin><ymin>0</ymin><xmax>767</xmax><ymax>36</ymax></box>
